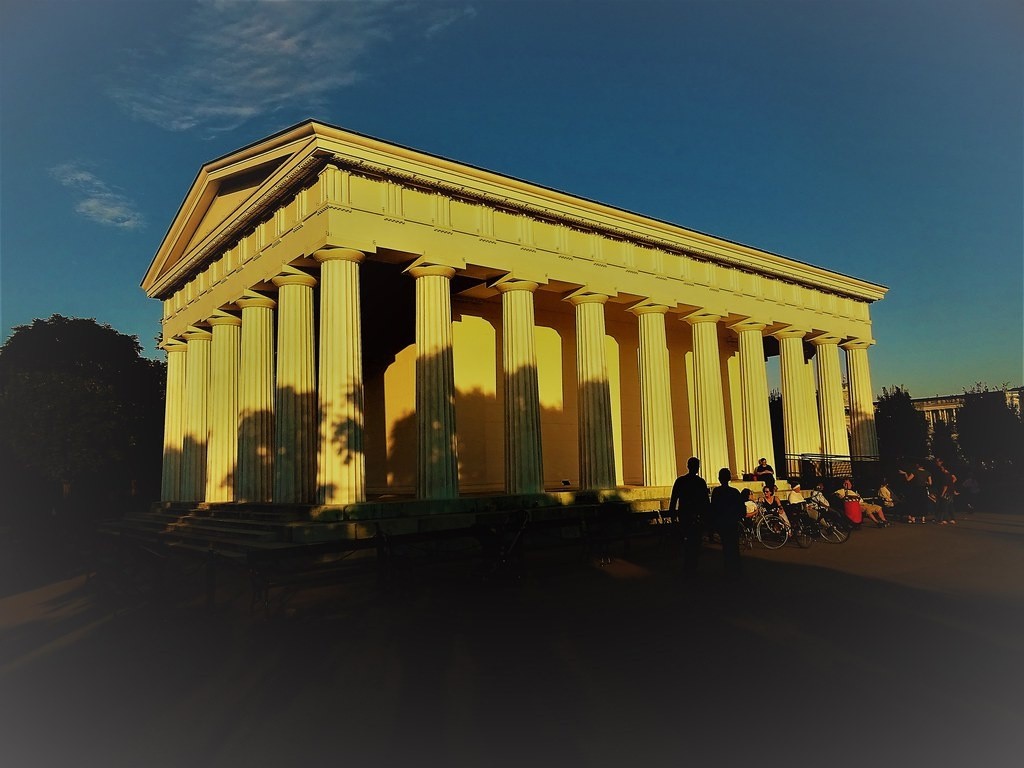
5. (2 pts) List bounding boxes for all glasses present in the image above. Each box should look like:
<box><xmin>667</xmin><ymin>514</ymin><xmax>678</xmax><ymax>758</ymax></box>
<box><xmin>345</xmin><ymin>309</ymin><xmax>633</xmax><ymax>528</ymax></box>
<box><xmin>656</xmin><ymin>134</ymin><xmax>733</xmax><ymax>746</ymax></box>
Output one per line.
<box><xmin>763</xmin><ymin>491</ymin><xmax>770</xmax><ymax>493</ymax></box>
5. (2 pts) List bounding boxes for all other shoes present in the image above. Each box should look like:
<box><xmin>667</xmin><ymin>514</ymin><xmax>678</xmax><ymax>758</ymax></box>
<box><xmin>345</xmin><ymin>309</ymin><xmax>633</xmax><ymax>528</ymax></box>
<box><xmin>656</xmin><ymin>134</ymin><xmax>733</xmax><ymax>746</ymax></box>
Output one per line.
<box><xmin>878</xmin><ymin>522</ymin><xmax>886</xmax><ymax>529</ymax></box>
<box><xmin>883</xmin><ymin>520</ymin><xmax>890</xmax><ymax>525</ymax></box>
<box><xmin>908</xmin><ymin>518</ymin><xmax>955</xmax><ymax>524</ymax></box>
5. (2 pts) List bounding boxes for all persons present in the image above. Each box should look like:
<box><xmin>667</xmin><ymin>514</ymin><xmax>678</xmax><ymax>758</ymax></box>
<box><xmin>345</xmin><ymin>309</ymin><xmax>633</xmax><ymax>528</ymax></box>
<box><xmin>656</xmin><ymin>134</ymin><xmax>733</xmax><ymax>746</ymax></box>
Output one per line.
<box><xmin>669</xmin><ymin>458</ymin><xmax>962</xmax><ymax>576</ymax></box>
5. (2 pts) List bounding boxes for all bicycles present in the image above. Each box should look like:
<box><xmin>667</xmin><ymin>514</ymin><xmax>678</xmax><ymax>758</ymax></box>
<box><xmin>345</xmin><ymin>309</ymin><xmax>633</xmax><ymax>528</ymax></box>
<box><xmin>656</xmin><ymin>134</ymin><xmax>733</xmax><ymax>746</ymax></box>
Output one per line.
<box><xmin>734</xmin><ymin>497</ymin><xmax>789</xmax><ymax>551</ymax></box>
<box><xmin>792</xmin><ymin>490</ymin><xmax>851</xmax><ymax>548</ymax></box>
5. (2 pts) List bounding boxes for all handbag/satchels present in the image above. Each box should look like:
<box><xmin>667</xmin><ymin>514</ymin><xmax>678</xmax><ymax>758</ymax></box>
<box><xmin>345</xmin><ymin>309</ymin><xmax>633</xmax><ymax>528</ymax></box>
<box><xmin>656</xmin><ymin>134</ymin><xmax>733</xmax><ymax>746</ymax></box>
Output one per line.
<box><xmin>742</xmin><ymin>473</ymin><xmax>756</xmax><ymax>481</ymax></box>
